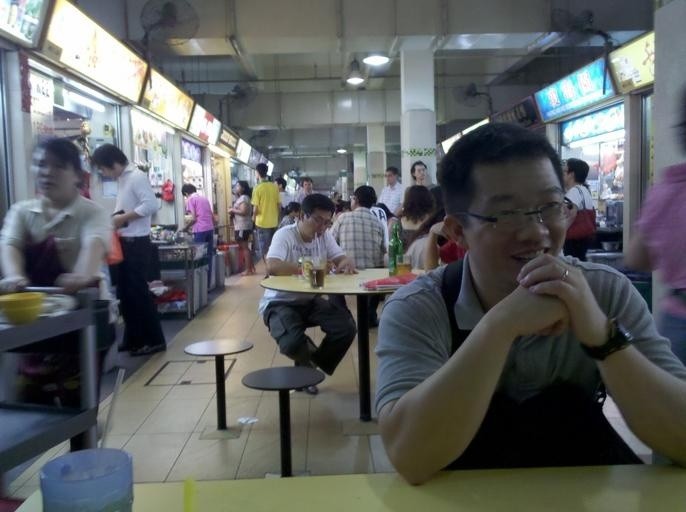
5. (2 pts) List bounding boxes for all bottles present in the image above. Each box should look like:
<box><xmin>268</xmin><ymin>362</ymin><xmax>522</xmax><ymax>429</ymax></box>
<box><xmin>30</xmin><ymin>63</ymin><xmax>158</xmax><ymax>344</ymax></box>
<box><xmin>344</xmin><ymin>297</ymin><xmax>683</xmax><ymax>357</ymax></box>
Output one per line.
<box><xmin>388</xmin><ymin>222</ymin><xmax>404</xmax><ymax>277</ymax></box>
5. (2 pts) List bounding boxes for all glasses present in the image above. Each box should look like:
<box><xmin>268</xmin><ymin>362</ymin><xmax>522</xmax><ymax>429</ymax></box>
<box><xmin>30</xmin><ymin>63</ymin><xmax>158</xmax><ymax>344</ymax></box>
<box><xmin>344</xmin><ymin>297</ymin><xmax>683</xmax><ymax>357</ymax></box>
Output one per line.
<box><xmin>454</xmin><ymin>196</ymin><xmax>579</xmax><ymax>235</ymax></box>
<box><xmin>304</xmin><ymin>209</ymin><xmax>333</xmax><ymax>228</ymax></box>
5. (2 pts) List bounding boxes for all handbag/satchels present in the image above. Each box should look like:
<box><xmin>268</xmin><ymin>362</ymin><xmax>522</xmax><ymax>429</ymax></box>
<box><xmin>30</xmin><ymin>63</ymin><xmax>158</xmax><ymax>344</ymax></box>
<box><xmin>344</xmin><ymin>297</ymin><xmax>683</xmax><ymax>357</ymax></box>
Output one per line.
<box><xmin>432</xmin><ymin>257</ymin><xmax>647</xmax><ymax>469</ymax></box>
<box><xmin>566</xmin><ymin>186</ymin><xmax>597</xmax><ymax>240</ymax></box>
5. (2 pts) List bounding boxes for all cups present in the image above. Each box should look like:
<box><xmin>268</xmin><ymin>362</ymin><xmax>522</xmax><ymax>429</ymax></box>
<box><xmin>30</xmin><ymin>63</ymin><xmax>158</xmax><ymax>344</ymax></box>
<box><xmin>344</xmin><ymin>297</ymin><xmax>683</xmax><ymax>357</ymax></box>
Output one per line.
<box><xmin>302</xmin><ymin>254</ymin><xmax>327</xmax><ymax>290</ymax></box>
<box><xmin>395</xmin><ymin>254</ymin><xmax>411</xmax><ymax>276</ymax></box>
<box><xmin>38</xmin><ymin>448</ymin><xmax>133</xmax><ymax>512</ymax></box>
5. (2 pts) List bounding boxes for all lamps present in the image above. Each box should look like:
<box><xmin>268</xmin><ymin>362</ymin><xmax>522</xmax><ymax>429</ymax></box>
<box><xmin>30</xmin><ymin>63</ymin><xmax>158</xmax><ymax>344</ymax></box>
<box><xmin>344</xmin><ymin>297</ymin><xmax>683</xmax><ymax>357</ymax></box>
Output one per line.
<box><xmin>361</xmin><ymin>51</ymin><xmax>389</xmax><ymax>65</ymax></box>
<box><xmin>344</xmin><ymin>52</ymin><xmax>365</xmax><ymax>86</ymax></box>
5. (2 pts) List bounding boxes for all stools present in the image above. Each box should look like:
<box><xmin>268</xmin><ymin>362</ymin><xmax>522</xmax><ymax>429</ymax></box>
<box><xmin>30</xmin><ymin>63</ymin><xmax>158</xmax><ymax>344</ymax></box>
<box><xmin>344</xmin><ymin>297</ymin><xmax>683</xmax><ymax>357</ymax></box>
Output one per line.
<box><xmin>240</xmin><ymin>366</ymin><xmax>324</xmax><ymax>478</ymax></box>
<box><xmin>183</xmin><ymin>336</ymin><xmax>256</xmax><ymax>445</ymax></box>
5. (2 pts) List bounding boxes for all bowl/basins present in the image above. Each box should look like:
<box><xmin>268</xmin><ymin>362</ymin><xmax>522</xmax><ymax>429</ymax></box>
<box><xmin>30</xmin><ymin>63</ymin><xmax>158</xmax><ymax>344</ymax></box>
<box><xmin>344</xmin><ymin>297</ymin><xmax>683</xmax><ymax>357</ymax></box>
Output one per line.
<box><xmin>0</xmin><ymin>292</ymin><xmax>47</xmax><ymax>325</ymax></box>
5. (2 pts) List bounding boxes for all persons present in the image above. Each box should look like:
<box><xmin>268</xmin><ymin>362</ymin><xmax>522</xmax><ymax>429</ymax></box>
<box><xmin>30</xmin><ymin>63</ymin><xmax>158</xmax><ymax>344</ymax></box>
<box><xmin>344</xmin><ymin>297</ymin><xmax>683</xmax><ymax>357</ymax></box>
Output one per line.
<box><xmin>92</xmin><ymin>144</ymin><xmax>166</xmax><ymax>356</ymax></box>
<box><xmin>0</xmin><ymin>139</ymin><xmax>115</xmax><ymax>452</ymax></box>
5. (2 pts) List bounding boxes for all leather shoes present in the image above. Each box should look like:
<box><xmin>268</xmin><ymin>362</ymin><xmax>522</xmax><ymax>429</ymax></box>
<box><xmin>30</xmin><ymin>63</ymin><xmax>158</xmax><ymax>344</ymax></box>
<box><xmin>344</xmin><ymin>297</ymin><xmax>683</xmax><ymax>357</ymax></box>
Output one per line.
<box><xmin>130</xmin><ymin>341</ymin><xmax>166</xmax><ymax>357</ymax></box>
<box><xmin>117</xmin><ymin>342</ymin><xmax>132</xmax><ymax>352</ymax></box>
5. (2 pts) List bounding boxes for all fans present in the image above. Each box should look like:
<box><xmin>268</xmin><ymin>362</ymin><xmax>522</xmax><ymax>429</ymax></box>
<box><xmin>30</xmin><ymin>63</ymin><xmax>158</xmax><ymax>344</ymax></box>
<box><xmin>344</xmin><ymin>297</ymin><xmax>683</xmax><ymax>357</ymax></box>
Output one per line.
<box><xmin>451</xmin><ymin>80</ymin><xmax>493</xmax><ymax>110</ymax></box>
<box><xmin>548</xmin><ymin>1</ymin><xmax>617</xmax><ymax>58</ymax></box>
<box><xmin>123</xmin><ymin>0</ymin><xmax>203</xmax><ymax>65</ymax></box>
<box><xmin>216</xmin><ymin>82</ymin><xmax>259</xmax><ymax>111</ymax></box>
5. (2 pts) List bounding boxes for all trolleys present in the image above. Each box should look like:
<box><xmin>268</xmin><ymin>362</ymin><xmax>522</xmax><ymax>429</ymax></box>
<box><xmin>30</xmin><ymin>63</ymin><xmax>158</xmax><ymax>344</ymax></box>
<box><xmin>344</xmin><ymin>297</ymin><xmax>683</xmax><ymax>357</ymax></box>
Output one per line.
<box><xmin>1</xmin><ymin>276</ymin><xmax>111</xmax><ymax>497</ymax></box>
